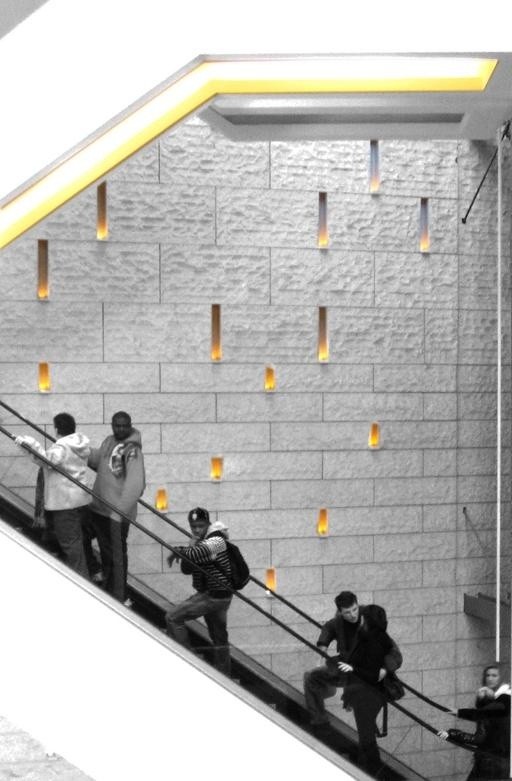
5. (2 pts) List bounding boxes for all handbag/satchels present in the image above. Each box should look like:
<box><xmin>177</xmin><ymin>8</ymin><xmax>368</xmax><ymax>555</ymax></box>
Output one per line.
<box><xmin>377</xmin><ymin>671</ymin><xmax>405</xmax><ymax>703</ymax></box>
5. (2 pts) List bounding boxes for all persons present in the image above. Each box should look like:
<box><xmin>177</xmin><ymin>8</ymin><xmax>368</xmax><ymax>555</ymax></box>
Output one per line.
<box><xmin>300</xmin><ymin>589</ymin><xmax>403</xmax><ymax>733</ymax></box>
<box><xmin>164</xmin><ymin>506</ymin><xmax>234</xmax><ymax>676</ymax></box>
<box><xmin>11</xmin><ymin>411</ymin><xmax>94</xmax><ymax>582</ymax></box>
<box><xmin>334</xmin><ymin>604</ymin><xmax>393</xmax><ymax>775</ymax></box>
<box><xmin>436</xmin><ymin>686</ymin><xmax>511</xmax><ymax>781</ymax></box>
<box><xmin>79</xmin><ymin>410</ymin><xmax>147</xmax><ymax>609</ymax></box>
<box><xmin>443</xmin><ymin>661</ymin><xmax>511</xmax><ymax>734</ymax></box>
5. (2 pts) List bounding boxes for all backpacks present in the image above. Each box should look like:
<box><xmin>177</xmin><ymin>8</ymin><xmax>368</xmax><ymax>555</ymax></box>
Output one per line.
<box><xmin>205</xmin><ymin>530</ymin><xmax>249</xmax><ymax>590</ymax></box>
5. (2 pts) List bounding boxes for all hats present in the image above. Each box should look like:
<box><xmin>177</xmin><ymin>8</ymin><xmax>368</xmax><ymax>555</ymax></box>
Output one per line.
<box><xmin>188</xmin><ymin>507</ymin><xmax>212</xmax><ymax>524</ymax></box>
